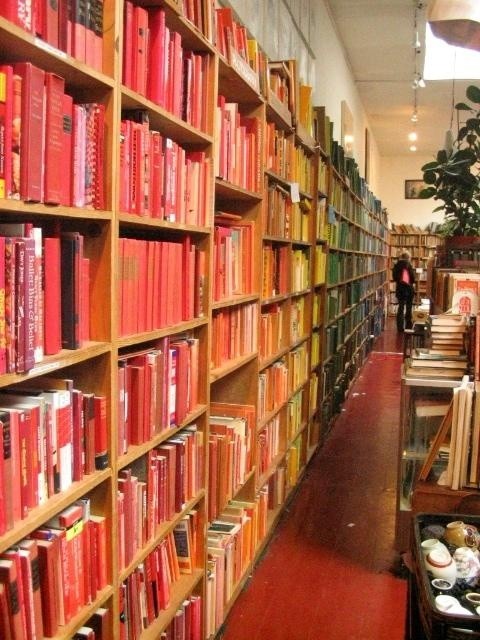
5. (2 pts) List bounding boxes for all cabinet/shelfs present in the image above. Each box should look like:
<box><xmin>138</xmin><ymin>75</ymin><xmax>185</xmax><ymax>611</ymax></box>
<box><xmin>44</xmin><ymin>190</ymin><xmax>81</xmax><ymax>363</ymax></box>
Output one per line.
<box><xmin>261</xmin><ymin>54</ymin><xmax>387</xmax><ymax>550</ymax></box>
<box><xmin>0</xmin><ymin>2</ymin><xmax>260</xmax><ymax>640</ymax></box>
<box><xmin>419</xmin><ymin>403</ymin><xmax>435</xmax><ymax>408</ymax></box>
<box><xmin>404</xmin><ymin>234</ymin><xmax>480</xmax><ymax>364</ymax></box>
<box><xmin>387</xmin><ymin>228</ymin><xmax>458</xmax><ymax>317</ymax></box>
<box><xmin>395</xmin><ymin>360</ymin><xmax>480</xmax><ymax>551</ymax></box>
<box><xmin>406</xmin><ymin>479</ymin><xmax>480</xmax><ymax>636</ymax></box>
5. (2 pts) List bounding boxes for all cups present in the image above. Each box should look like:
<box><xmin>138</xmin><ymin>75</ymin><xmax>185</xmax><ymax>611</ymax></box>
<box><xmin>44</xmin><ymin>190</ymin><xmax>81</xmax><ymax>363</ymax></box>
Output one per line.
<box><xmin>466</xmin><ymin>593</ymin><xmax>480</xmax><ymax>604</ymax></box>
<box><xmin>430</xmin><ymin>579</ymin><xmax>454</xmax><ymax>590</ymax></box>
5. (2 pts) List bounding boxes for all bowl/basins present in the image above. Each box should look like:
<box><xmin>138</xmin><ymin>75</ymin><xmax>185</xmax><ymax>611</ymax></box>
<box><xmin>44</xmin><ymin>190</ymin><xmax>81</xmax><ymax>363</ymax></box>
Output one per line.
<box><xmin>425</xmin><ymin>551</ymin><xmax>457</xmax><ymax>585</ymax></box>
<box><xmin>421</xmin><ymin>539</ymin><xmax>449</xmax><ymax>554</ymax></box>
<box><xmin>443</xmin><ymin>521</ymin><xmax>479</xmax><ymax>554</ymax></box>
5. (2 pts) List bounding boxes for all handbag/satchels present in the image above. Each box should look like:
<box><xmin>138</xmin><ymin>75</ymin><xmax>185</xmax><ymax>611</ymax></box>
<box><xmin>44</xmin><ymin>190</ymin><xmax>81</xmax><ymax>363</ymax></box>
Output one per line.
<box><xmin>400</xmin><ymin>263</ymin><xmax>411</xmax><ymax>286</ymax></box>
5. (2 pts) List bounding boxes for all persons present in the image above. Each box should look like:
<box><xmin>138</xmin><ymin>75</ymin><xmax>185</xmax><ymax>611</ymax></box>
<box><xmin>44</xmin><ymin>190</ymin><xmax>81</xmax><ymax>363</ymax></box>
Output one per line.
<box><xmin>392</xmin><ymin>251</ymin><xmax>417</xmax><ymax>332</ymax></box>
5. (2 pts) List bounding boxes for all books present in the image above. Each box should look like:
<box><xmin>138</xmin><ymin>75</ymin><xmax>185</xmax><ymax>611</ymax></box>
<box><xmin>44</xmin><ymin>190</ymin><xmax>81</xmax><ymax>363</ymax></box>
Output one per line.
<box><xmin>293</xmin><ymin>144</ymin><xmax>312</xmax><ymax>197</ymax></box>
<box><xmin>284</xmin><ymin>390</ymin><xmax>305</xmax><ymax>447</ymax></box>
<box><xmin>418</xmin><ymin>384</ymin><xmax>480</xmax><ymax>491</ymax></box>
<box><xmin>259</xmin><ymin>412</ymin><xmax>281</xmax><ymax>477</ymax></box>
<box><xmin>121</xmin><ymin>510</ymin><xmax>197</xmax><ymax>640</ymax></box>
<box><xmin>0</xmin><ymin>378</ymin><xmax>107</xmax><ymax>532</ymax></box>
<box><xmin>117</xmin><ymin>330</ymin><xmax>199</xmax><ymax>457</ymax></box>
<box><xmin>215</xmin><ymin>3</ymin><xmax>268</xmax><ymax>102</ymax></box>
<box><xmin>280</xmin><ymin>75</ymin><xmax>316</xmax><ymax>142</ymax></box>
<box><xmin>284</xmin><ymin>248</ymin><xmax>311</xmax><ymax>295</ymax></box>
<box><xmin>290</xmin><ymin>343</ymin><xmax>310</xmax><ymax>399</ymax></box>
<box><xmin>263</xmin><ymin>242</ymin><xmax>288</xmax><ymax>300</ymax></box>
<box><xmin>260</xmin><ymin>354</ymin><xmax>291</xmax><ymax>425</ymax></box>
<box><xmin>267</xmin><ymin>182</ymin><xmax>283</xmax><ymax>231</ymax></box>
<box><xmin>402</xmin><ymin>223</ymin><xmax>447</xmax><ymax>269</ymax></box>
<box><xmin>402</xmin><ymin>268</ymin><xmax>480</xmax><ymax>377</ymax></box>
<box><xmin>286</xmin><ymin>297</ymin><xmax>306</xmax><ymax>348</ymax></box>
<box><xmin>256</xmin><ymin>463</ymin><xmax>289</xmax><ymax>544</ymax></box>
<box><xmin>118</xmin><ymin>233</ymin><xmax>206</xmax><ymax>340</ymax></box>
<box><xmin>0</xmin><ymin>499</ymin><xmax>107</xmax><ymax>640</ymax></box>
<box><xmin>0</xmin><ymin>0</ymin><xmax>104</xmax><ymax>71</ymax></box>
<box><xmin>212</xmin><ymin>303</ymin><xmax>259</xmax><ymax>372</ymax></box>
<box><xmin>0</xmin><ymin>59</ymin><xmax>104</xmax><ymax>204</ymax></box>
<box><xmin>162</xmin><ymin>594</ymin><xmax>204</xmax><ymax>640</ymax></box>
<box><xmin>0</xmin><ymin>223</ymin><xmax>90</xmax><ymax>378</ymax></box>
<box><xmin>120</xmin><ymin>113</ymin><xmax>209</xmax><ymax>227</ymax></box>
<box><xmin>216</xmin><ymin>91</ymin><xmax>264</xmax><ymax>198</ymax></box>
<box><xmin>259</xmin><ymin>304</ymin><xmax>285</xmax><ymax>363</ymax></box>
<box><xmin>285</xmin><ymin>433</ymin><xmax>304</xmax><ymax>500</ymax></box>
<box><xmin>287</xmin><ymin>192</ymin><xmax>311</xmax><ymax>245</ymax></box>
<box><xmin>118</xmin><ymin>423</ymin><xmax>204</xmax><ymax>577</ymax></box>
<box><xmin>302</xmin><ymin>103</ymin><xmax>401</xmax><ymax>437</ymax></box>
<box><xmin>266</xmin><ymin>116</ymin><xmax>293</xmax><ymax>186</ymax></box>
<box><xmin>269</xmin><ymin>59</ymin><xmax>292</xmax><ymax>111</ymax></box>
<box><xmin>215</xmin><ymin>211</ymin><xmax>253</xmax><ymax>303</ymax></box>
<box><xmin>209</xmin><ymin>399</ymin><xmax>256</xmax><ymax>520</ymax></box>
<box><xmin>124</xmin><ymin>2</ymin><xmax>210</xmax><ymax>140</ymax></box>
<box><xmin>205</xmin><ymin>500</ymin><xmax>260</xmax><ymax>638</ymax></box>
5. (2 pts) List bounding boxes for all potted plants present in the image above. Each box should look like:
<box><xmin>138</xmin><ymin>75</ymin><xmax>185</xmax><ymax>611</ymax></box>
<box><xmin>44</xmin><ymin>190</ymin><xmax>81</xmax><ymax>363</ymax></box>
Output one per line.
<box><xmin>416</xmin><ymin>84</ymin><xmax>480</xmax><ymax>248</ymax></box>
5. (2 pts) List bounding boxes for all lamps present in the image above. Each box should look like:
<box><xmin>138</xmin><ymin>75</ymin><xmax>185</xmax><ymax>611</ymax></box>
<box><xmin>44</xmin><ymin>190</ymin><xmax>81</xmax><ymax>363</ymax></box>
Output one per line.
<box><xmin>409</xmin><ymin>113</ymin><xmax>421</xmax><ymax>123</ymax></box>
<box><xmin>413</xmin><ymin>31</ymin><xmax>420</xmax><ymax>48</ymax></box>
<box><xmin>422</xmin><ymin>21</ymin><xmax>480</xmax><ymax>80</ymax></box>
<box><xmin>412</xmin><ymin>75</ymin><xmax>425</xmax><ymax>89</ymax></box>
